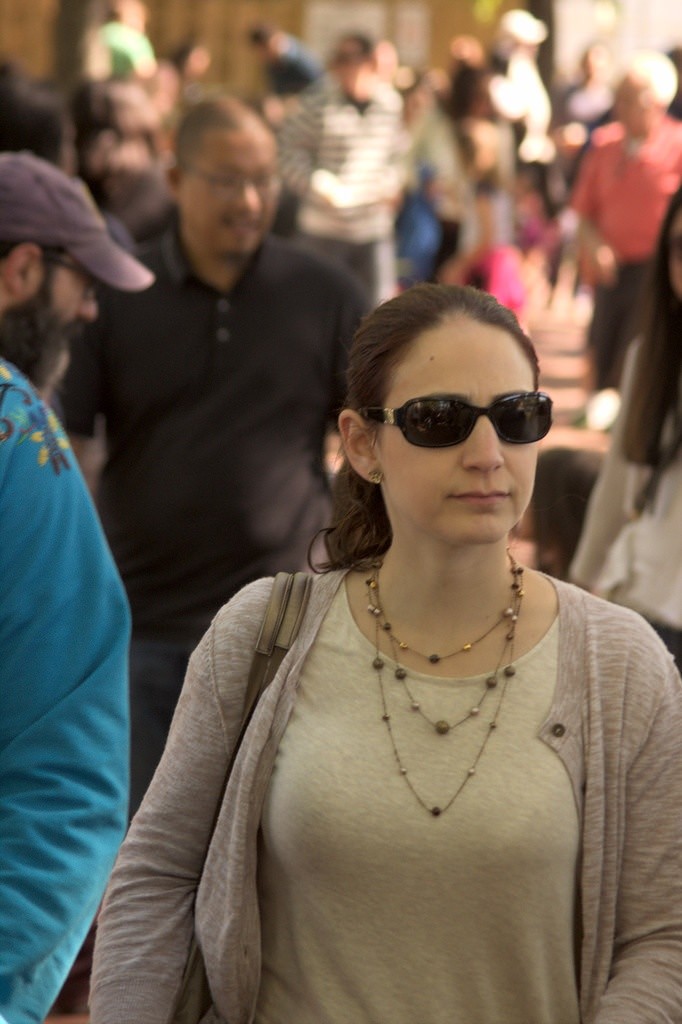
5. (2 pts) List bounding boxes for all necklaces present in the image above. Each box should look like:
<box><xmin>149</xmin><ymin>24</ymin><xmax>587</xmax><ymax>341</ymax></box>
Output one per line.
<box><xmin>366</xmin><ymin>548</ymin><xmax>525</xmax><ymax>817</ymax></box>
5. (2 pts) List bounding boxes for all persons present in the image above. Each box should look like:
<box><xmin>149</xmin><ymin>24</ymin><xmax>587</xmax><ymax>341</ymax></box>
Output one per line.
<box><xmin>56</xmin><ymin>99</ymin><xmax>370</xmax><ymax>822</ymax></box>
<box><xmin>86</xmin><ymin>282</ymin><xmax>682</xmax><ymax>1024</ymax></box>
<box><xmin>0</xmin><ymin>0</ymin><xmax>682</xmax><ymax>398</ymax></box>
<box><xmin>568</xmin><ymin>186</ymin><xmax>682</xmax><ymax>681</ymax></box>
<box><xmin>1</xmin><ymin>362</ymin><xmax>132</xmax><ymax>1024</ymax></box>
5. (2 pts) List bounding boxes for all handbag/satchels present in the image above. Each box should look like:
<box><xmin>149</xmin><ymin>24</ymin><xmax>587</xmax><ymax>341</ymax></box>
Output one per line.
<box><xmin>159</xmin><ymin>572</ymin><xmax>313</xmax><ymax>1024</ymax></box>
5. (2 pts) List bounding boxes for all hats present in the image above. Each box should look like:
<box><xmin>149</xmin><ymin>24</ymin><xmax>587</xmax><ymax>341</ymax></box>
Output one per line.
<box><xmin>0</xmin><ymin>150</ymin><xmax>155</xmax><ymax>293</ymax></box>
<box><xmin>502</xmin><ymin>9</ymin><xmax>551</xmax><ymax>45</ymax></box>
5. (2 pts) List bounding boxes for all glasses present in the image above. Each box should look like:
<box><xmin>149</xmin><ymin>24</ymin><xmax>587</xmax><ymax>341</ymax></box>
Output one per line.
<box><xmin>360</xmin><ymin>391</ymin><xmax>554</xmax><ymax>448</ymax></box>
